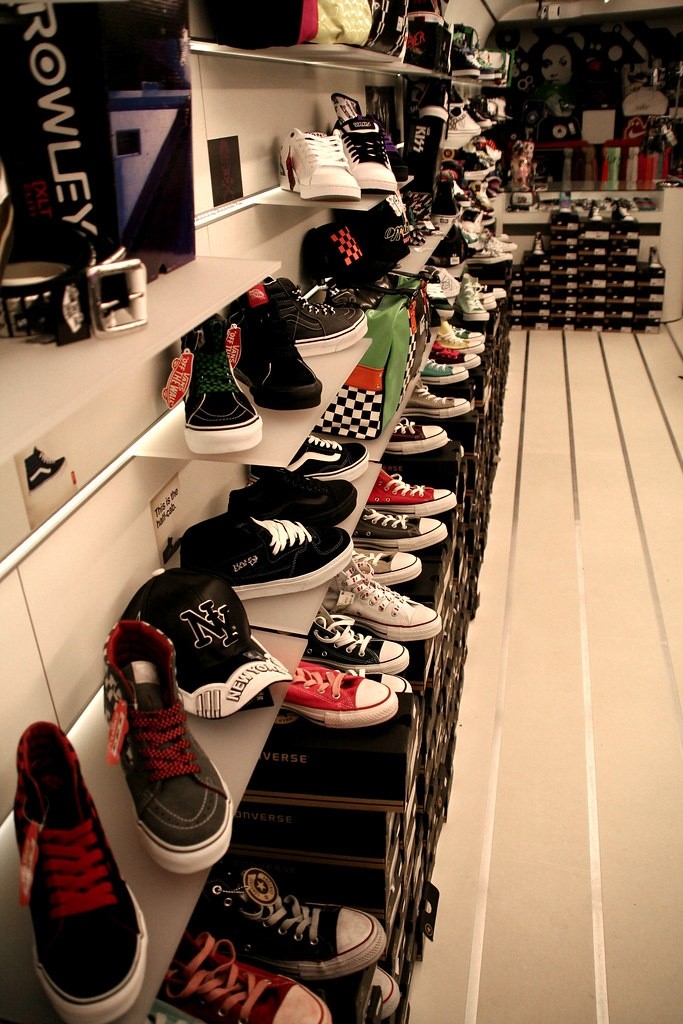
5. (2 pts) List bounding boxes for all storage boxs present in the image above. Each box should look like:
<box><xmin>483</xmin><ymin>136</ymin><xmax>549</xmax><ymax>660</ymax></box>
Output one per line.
<box><xmin>202</xmin><ymin>213</ymin><xmax>665</xmax><ymax>1024</ymax></box>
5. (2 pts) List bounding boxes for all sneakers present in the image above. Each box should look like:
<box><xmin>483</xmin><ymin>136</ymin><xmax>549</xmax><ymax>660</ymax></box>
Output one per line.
<box><xmin>0</xmin><ymin>0</ymin><xmax>683</xmax><ymax>1024</ymax></box>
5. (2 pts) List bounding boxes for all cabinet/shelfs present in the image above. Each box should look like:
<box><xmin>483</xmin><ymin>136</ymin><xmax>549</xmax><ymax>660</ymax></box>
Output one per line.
<box><xmin>1</xmin><ymin>43</ymin><xmax>484</xmax><ymax>1024</ymax></box>
